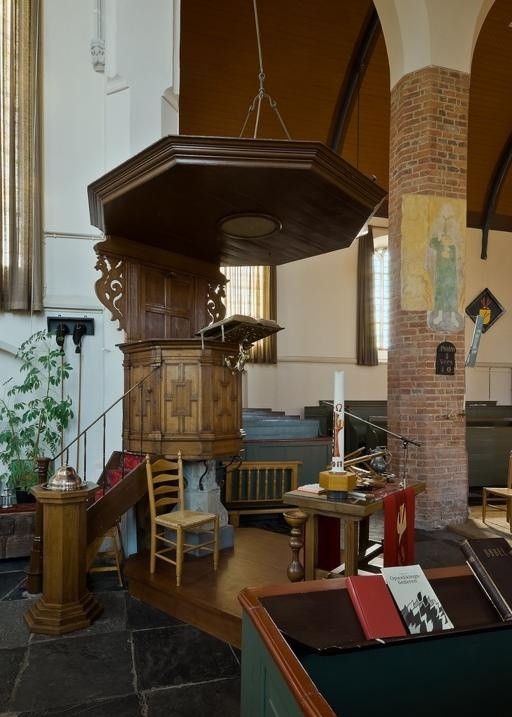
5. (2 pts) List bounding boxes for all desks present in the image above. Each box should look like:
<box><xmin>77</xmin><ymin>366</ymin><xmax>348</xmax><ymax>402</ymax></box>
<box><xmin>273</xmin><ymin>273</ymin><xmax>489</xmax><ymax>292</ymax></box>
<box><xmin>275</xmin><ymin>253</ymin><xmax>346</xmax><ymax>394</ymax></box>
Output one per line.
<box><xmin>282</xmin><ymin>478</ymin><xmax>426</xmax><ymax>581</ymax></box>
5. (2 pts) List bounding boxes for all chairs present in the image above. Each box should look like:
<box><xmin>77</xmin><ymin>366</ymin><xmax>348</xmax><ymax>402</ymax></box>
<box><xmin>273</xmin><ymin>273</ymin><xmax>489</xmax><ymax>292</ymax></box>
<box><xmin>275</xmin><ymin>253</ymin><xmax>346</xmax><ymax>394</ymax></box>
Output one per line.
<box><xmin>145</xmin><ymin>448</ymin><xmax>219</xmax><ymax>587</ymax></box>
<box><xmin>481</xmin><ymin>451</ymin><xmax>512</xmax><ymax>532</ymax></box>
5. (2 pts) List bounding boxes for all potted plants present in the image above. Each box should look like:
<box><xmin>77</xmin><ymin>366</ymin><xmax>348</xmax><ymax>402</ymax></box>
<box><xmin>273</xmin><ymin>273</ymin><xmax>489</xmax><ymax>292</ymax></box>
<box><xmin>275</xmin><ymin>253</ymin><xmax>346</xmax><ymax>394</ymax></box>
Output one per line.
<box><xmin>0</xmin><ymin>329</ymin><xmax>75</xmax><ymax>502</ymax></box>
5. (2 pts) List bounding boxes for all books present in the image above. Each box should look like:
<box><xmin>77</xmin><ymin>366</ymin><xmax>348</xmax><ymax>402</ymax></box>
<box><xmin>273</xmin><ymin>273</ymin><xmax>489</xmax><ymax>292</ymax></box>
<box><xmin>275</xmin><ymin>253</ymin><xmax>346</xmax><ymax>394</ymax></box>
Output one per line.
<box><xmin>297</xmin><ymin>483</ymin><xmax>327</xmax><ymax>494</ymax></box>
<box><xmin>344</xmin><ymin>574</ymin><xmax>408</xmax><ymax>640</ymax></box>
<box><xmin>379</xmin><ymin>561</ymin><xmax>454</xmax><ymax>636</ymax></box>
<box><xmin>458</xmin><ymin>535</ymin><xmax>512</xmax><ymax>623</ymax></box>
<box><xmin>193</xmin><ymin>314</ymin><xmax>286</xmax><ymax>338</ymax></box>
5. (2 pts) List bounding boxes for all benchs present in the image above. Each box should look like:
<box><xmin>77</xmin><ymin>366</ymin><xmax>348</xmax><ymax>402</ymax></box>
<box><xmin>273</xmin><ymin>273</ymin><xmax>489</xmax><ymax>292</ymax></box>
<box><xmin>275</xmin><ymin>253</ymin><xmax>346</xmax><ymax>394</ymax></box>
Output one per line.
<box><xmin>222</xmin><ymin>459</ymin><xmax>304</xmax><ymax>527</ymax></box>
<box><xmin>241</xmin><ymin>399</ymin><xmax>512</xmax><ymax>489</ymax></box>
